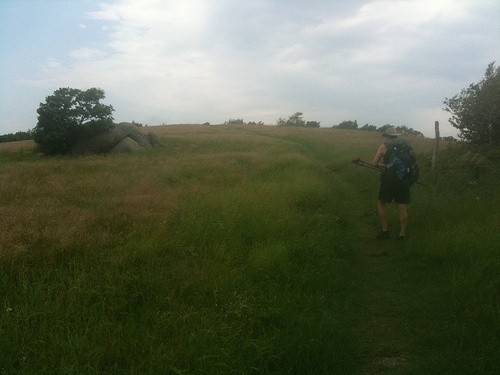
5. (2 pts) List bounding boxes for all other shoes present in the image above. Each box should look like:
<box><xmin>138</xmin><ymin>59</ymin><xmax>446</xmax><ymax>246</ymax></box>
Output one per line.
<box><xmin>375</xmin><ymin>230</ymin><xmax>389</xmax><ymax>239</ymax></box>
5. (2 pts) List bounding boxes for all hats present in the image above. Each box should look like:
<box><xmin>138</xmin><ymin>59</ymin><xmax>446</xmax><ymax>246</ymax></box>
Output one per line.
<box><xmin>381</xmin><ymin>126</ymin><xmax>401</xmax><ymax>137</ymax></box>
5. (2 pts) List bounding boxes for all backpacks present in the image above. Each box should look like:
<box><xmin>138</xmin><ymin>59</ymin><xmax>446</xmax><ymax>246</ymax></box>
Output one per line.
<box><xmin>384</xmin><ymin>140</ymin><xmax>419</xmax><ymax>182</ymax></box>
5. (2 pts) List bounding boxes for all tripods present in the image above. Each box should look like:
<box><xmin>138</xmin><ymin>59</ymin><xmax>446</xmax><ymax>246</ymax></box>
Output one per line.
<box><xmin>356</xmin><ymin>160</ymin><xmax>429</xmax><ymax>186</ymax></box>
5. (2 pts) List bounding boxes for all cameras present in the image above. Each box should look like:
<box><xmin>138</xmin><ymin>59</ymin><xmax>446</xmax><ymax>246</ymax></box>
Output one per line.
<box><xmin>352</xmin><ymin>156</ymin><xmax>361</xmax><ymax>164</ymax></box>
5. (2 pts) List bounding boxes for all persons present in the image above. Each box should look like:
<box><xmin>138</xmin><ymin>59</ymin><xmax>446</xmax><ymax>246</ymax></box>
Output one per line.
<box><xmin>371</xmin><ymin>126</ymin><xmax>411</xmax><ymax>241</ymax></box>
